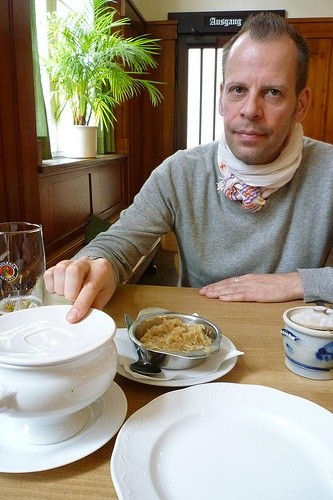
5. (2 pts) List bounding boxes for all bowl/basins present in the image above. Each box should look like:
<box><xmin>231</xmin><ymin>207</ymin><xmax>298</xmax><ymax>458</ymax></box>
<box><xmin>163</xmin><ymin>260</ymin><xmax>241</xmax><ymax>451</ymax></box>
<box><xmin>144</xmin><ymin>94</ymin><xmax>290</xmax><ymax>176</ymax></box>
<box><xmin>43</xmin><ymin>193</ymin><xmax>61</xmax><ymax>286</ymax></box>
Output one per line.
<box><xmin>129</xmin><ymin>307</ymin><xmax>222</xmax><ymax>370</ymax></box>
<box><xmin>279</xmin><ymin>306</ymin><xmax>333</xmax><ymax>380</ymax></box>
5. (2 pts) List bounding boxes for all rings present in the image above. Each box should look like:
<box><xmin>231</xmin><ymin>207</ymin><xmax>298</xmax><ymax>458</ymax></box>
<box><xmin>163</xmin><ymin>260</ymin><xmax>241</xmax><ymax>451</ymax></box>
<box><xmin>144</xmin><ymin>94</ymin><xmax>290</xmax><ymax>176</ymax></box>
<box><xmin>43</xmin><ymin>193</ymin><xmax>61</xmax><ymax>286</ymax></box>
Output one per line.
<box><xmin>234</xmin><ymin>277</ymin><xmax>241</xmax><ymax>282</ymax></box>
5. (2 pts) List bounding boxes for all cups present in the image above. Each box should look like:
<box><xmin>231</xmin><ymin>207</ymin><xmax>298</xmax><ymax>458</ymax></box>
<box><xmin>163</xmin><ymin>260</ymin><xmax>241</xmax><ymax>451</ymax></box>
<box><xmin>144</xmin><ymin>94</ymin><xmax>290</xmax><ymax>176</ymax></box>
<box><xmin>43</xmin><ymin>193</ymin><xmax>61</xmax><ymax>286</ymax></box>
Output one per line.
<box><xmin>0</xmin><ymin>221</ymin><xmax>46</xmax><ymax>317</ymax></box>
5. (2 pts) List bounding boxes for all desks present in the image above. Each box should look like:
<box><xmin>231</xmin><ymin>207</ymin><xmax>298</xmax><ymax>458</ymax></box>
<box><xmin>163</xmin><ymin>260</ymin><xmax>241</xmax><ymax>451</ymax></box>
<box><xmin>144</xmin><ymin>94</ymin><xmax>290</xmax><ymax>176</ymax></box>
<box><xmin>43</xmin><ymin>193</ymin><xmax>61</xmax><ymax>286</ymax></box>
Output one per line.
<box><xmin>0</xmin><ymin>283</ymin><xmax>333</xmax><ymax>500</ymax></box>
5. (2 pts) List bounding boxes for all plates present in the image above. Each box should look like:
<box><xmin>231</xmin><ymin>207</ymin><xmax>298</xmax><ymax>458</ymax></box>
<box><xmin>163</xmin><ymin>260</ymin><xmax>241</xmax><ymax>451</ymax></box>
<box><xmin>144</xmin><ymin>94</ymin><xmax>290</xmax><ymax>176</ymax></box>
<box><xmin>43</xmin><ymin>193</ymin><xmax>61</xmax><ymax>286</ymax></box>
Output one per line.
<box><xmin>0</xmin><ymin>305</ymin><xmax>116</xmax><ymax>366</ymax></box>
<box><xmin>109</xmin><ymin>382</ymin><xmax>333</xmax><ymax>500</ymax></box>
<box><xmin>0</xmin><ymin>380</ymin><xmax>128</xmax><ymax>473</ymax></box>
<box><xmin>113</xmin><ymin>328</ymin><xmax>237</xmax><ymax>387</ymax></box>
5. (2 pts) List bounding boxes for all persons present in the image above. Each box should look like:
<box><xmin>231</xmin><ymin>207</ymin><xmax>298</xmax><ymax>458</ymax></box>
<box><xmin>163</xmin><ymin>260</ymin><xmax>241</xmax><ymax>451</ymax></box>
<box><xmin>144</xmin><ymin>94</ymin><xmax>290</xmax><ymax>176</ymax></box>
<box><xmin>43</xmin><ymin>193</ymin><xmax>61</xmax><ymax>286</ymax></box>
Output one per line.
<box><xmin>45</xmin><ymin>11</ymin><xmax>333</xmax><ymax>323</ymax></box>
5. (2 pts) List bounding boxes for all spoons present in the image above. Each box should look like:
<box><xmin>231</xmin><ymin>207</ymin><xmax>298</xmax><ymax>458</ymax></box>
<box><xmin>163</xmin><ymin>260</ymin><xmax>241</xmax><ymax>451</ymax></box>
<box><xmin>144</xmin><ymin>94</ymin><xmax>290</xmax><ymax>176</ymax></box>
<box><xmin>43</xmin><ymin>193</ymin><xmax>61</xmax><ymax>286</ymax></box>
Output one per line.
<box><xmin>123</xmin><ymin>313</ymin><xmax>162</xmax><ymax>375</ymax></box>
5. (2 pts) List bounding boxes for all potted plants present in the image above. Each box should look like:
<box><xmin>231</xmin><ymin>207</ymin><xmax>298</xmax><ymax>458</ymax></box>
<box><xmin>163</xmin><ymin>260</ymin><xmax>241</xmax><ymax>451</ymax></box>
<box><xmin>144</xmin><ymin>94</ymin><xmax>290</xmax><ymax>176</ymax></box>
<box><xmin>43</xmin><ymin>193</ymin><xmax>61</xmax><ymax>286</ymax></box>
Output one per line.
<box><xmin>39</xmin><ymin>0</ymin><xmax>166</xmax><ymax>159</ymax></box>
